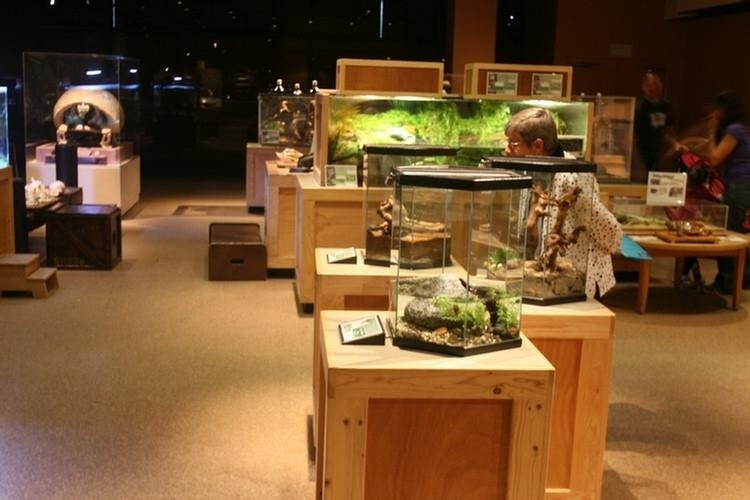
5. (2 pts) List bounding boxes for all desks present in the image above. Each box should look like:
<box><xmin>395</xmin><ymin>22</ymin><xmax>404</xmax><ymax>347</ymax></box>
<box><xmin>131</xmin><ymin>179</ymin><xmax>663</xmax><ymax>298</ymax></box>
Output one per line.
<box><xmin>620</xmin><ymin>227</ymin><xmax>750</xmax><ymax>314</ymax></box>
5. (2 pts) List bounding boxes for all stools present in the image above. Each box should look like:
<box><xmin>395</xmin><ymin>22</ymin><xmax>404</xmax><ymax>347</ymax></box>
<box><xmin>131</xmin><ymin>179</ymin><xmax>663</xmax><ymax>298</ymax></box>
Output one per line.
<box><xmin>208</xmin><ymin>219</ymin><xmax>268</xmax><ymax>281</ymax></box>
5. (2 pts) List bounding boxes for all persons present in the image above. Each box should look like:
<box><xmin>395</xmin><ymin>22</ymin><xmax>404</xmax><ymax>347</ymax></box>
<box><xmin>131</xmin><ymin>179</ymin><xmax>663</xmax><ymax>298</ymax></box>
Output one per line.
<box><xmin>503</xmin><ymin>106</ymin><xmax>622</xmax><ymax>303</ymax></box>
<box><xmin>633</xmin><ymin>70</ymin><xmax>690</xmax><ymax>172</ymax></box>
<box><xmin>705</xmin><ymin>89</ymin><xmax>750</xmax><ymax>297</ymax></box>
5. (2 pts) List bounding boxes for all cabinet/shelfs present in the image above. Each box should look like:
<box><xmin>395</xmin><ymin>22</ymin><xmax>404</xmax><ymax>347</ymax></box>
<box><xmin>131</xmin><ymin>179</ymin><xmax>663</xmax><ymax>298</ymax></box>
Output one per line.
<box><xmin>46</xmin><ymin>203</ymin><xmax>124</xmax><ymax>270</ymax></box>
<box><xmin>502</xmin><ymin>296</ymin><xmax>616</xmax><ymax>499</ymax></box>
<box><xmin>318</xmin><ymin>308</ymin><xmax>555</xmax><ymax>500</ymax></box>
<box><xmin>313</xmin><ymin>242</ymin><xmax>457</xmax><ymax>459</ymax></box>
